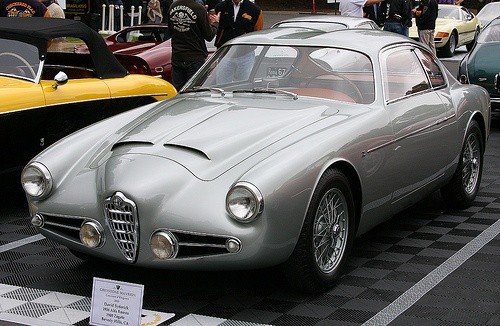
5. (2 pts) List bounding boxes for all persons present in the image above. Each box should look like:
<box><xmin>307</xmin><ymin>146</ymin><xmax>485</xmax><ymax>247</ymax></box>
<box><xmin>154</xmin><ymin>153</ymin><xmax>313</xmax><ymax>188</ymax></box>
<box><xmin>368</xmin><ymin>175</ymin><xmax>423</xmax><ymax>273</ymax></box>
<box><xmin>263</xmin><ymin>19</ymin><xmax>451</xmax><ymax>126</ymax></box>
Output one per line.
<box><xmin>0</xmin><ymin>0</ymin><xmax>66</xmax><ymax>52</ymax></box>
<box><xmin>339</xmin><ymin>0</ymin><xmax>438</xmax><ymax>58</ymax></box>
<box><xmin>167</xmin><ymin>0</ymin><xmax>264</xmax><ymax>93</ymax></box>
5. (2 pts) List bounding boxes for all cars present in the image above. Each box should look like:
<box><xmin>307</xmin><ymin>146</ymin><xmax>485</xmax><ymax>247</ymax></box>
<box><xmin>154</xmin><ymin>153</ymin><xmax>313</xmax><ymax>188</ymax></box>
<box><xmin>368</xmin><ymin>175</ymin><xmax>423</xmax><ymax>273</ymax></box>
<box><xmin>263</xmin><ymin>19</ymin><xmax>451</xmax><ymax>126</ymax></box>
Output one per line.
<box><xmin>22</xmin><ymin>27</ymin><xmax>491</xmax><ymax>292</ymax></box>
<box><xmin>272</xmin><ymin>15</ymin><xmax>381</xmax><ymax>32</ymax></box>
<box><xmin>402</xmin><ymin>0</ymin><xmax>500</xmax><ymax>133</ymax></box>
<box><xmin>0</xmin><ymin>16</ymin><xmax>216</xmax><ymax>210</ymax></box>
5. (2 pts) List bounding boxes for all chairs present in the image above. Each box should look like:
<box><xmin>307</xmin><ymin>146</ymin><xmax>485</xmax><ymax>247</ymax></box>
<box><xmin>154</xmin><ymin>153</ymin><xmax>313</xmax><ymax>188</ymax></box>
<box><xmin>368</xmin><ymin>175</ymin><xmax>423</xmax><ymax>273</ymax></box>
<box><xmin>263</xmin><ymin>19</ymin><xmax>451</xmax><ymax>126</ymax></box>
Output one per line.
<box><xmin>138</xmin><ymin>35</ymin><xmax>156</xmax><ymax>41</ymax></box>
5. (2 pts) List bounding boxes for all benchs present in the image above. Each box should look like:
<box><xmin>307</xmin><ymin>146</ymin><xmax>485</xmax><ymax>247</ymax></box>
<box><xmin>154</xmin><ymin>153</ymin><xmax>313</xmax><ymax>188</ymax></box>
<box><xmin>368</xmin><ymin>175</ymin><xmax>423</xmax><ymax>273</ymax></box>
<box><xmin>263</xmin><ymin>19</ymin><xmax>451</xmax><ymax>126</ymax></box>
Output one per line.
<box><xmin>17</xmin><ymin>65</ymin><xmax>95</xmax><ymax>79</ymax></box>
